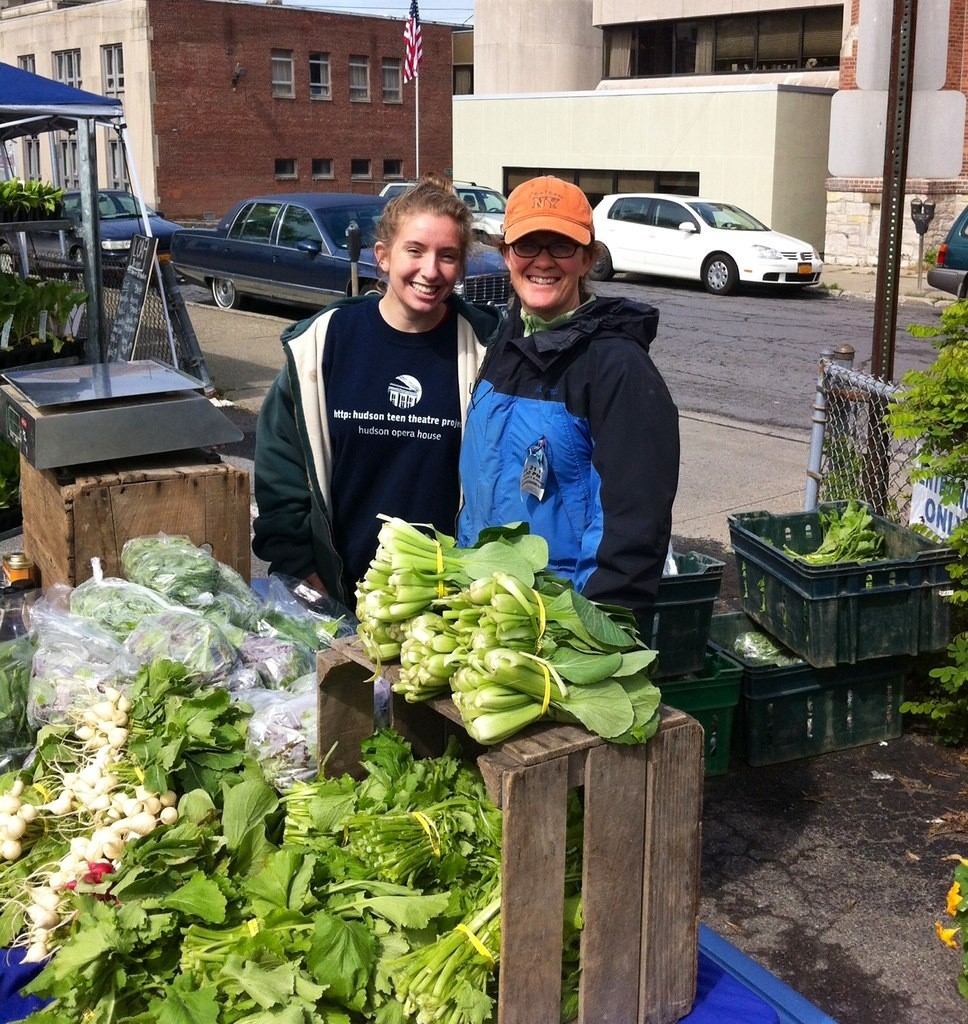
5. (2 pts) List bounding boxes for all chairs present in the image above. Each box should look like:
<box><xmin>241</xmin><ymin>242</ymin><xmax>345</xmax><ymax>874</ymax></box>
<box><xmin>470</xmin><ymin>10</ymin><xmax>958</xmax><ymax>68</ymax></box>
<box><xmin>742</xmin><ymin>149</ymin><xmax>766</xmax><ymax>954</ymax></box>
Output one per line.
<box><xmin>463</xmin><ymin>194</ymin><xmax>476</xmax><ymax>208</ymax></box>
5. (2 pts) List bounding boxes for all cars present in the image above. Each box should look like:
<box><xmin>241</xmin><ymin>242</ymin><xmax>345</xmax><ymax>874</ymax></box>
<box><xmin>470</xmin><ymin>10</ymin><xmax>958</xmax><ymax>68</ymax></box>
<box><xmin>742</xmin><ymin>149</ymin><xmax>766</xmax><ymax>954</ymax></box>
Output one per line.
<box><xmin>172</xmin><ymin>193</ymin><xmax>512</xmax><ymax>309</ymax></box>
<box><xmin>0</xmin><ymin>189</ymin><xmax>193</xmax><ymax>285</ymax></box>
<box><xmin>590</xmin><ymin>192</ymin><xmax>825</xmax><ymax>295</ymax></box>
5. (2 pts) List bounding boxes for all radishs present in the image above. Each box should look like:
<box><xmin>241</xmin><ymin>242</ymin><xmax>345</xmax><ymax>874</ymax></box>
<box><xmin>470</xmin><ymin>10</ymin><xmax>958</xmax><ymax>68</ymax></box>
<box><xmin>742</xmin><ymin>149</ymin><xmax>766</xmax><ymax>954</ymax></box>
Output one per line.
<box><xmin>0</xmin><ymin>684</ymin><xmax>181</xmax><ymax>964</ymax></box>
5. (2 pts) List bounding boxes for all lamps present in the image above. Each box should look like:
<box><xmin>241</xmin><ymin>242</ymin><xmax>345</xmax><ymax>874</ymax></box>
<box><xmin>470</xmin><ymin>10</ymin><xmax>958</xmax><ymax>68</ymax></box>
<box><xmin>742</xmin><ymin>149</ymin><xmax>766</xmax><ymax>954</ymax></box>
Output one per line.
<box><xmin>232</xmin><ymin>62</ymin><xmax>247</xmax><ymax>93</ymax></box>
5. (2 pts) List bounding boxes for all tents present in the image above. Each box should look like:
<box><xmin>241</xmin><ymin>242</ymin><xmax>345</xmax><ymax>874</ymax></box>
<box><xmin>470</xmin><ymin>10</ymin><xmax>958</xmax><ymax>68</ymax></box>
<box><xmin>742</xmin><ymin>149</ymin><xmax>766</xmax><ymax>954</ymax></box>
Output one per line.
<box><xmin>1</xmin><ymin>61</ymin><xmax>179</xmax><ymax>366</ymax></box>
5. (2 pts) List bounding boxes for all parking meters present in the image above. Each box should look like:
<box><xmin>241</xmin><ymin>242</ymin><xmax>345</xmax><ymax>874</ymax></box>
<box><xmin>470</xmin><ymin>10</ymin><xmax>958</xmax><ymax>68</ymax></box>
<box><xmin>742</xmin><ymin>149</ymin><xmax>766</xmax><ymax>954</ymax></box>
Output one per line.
<box><xmin>910</xmin><ymin>198</ymin><xmax>935</xmax><ymax>291</ymax></box>
<box><xmin>344</xmin><ymin>222</ymin><xmax>362</xmax><ymax>300</ymax></box>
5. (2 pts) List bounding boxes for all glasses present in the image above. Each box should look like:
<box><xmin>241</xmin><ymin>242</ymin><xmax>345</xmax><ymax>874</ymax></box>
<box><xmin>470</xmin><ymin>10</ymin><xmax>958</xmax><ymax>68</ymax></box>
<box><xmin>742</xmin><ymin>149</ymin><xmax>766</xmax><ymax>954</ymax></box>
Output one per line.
<box><xmin>511</xmin><ymin>239</ymin><xmax>582</xmax><ymax>259</ymax></box>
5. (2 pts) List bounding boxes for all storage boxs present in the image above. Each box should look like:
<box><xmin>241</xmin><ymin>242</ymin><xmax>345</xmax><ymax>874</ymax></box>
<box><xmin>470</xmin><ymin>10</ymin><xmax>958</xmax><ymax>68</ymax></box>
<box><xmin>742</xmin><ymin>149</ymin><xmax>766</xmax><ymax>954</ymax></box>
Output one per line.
<box><xmin>20</xmin><ymin>452</ymin><xmax>252</xmax><ymax>611</ymax></box>
<box><xmin>315</xmin><ymin>498</ymin><xmax>958</xmax><ymax>1024</ymax></box>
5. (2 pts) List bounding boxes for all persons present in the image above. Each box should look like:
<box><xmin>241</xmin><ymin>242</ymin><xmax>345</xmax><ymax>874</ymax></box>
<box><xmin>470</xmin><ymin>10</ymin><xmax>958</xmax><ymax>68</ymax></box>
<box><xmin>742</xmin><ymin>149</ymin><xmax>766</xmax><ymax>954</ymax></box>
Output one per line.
<box><xmin>252</xmin><ymin>174</ymin><xmax>502</xmax><ymax>617</ymax></box>
<box><xmin>459</xmin><ymin>175</ymin><xmax>681</xmax><ymax>614</ymax></box>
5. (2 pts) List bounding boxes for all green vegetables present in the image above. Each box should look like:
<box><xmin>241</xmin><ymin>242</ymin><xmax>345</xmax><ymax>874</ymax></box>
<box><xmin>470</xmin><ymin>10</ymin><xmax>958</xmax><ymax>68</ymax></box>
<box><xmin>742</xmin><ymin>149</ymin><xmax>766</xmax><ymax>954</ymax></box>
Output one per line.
<box><xmin>0</xmin><ymin>531</ymin><xmax>344</xmax><ymax>791</ymax></box>
<box><xmin>356</xmin><ymin>514</ymin><xmax>662</xmax><ymax>747</ymax></box>
<box><xmin>119</xmin><ymin>730</ymin><xmax>594</xmax><ymax>1024</ymax></box>
<box><xmin>732</xmin><ymin>499</ymin><xmax>886</xmax><ymax>671</ymax></box>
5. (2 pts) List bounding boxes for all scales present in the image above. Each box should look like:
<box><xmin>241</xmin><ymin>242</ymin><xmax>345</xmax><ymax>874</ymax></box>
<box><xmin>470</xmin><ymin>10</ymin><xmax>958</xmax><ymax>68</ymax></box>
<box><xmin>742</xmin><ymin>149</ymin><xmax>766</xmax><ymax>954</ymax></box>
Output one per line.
<box><xmin>0</xmin><ymin>355</ymin><xmax>246</xmax><ymax>486</ymax></box>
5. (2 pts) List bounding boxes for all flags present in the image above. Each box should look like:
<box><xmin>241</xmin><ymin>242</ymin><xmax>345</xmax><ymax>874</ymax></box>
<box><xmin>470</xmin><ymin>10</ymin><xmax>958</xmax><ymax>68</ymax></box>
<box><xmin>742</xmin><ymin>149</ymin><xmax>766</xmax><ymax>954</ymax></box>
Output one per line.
<box><xmin>403</xmin><ymin>0</ymin><xmax>423</xmax><ymax>86</ymax></box>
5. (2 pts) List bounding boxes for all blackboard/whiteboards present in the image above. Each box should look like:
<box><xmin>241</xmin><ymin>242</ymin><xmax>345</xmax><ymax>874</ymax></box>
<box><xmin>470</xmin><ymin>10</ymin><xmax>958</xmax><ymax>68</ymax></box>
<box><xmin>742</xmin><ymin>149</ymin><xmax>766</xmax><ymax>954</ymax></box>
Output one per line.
<box><xmin>106</xmin><ymin>234</ymin><xmax>158</xmax><ymax>362</ymax></box>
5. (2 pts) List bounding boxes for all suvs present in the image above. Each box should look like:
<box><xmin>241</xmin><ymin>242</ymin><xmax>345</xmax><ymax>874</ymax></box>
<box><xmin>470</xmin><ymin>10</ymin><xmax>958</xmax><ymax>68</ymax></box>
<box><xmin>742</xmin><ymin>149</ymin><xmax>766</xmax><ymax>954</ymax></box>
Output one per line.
<box><xmin>375</xmin><ymin>179</ymin><xmax>510</xmax><ymax>248</ymax></box>
<box><xmin>927</xmin><ymin>205</ymin><xmax>968</xmax><ymax>301</ymax></box>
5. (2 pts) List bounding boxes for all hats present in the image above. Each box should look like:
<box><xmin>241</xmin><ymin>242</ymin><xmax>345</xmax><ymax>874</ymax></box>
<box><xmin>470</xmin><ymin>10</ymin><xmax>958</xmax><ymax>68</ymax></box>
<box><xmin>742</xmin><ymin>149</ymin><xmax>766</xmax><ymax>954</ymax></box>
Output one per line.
<box><xmin>502</xmin><ymin>175</ymin><xmax>595</xmax><ymax>246</ymax></box>
